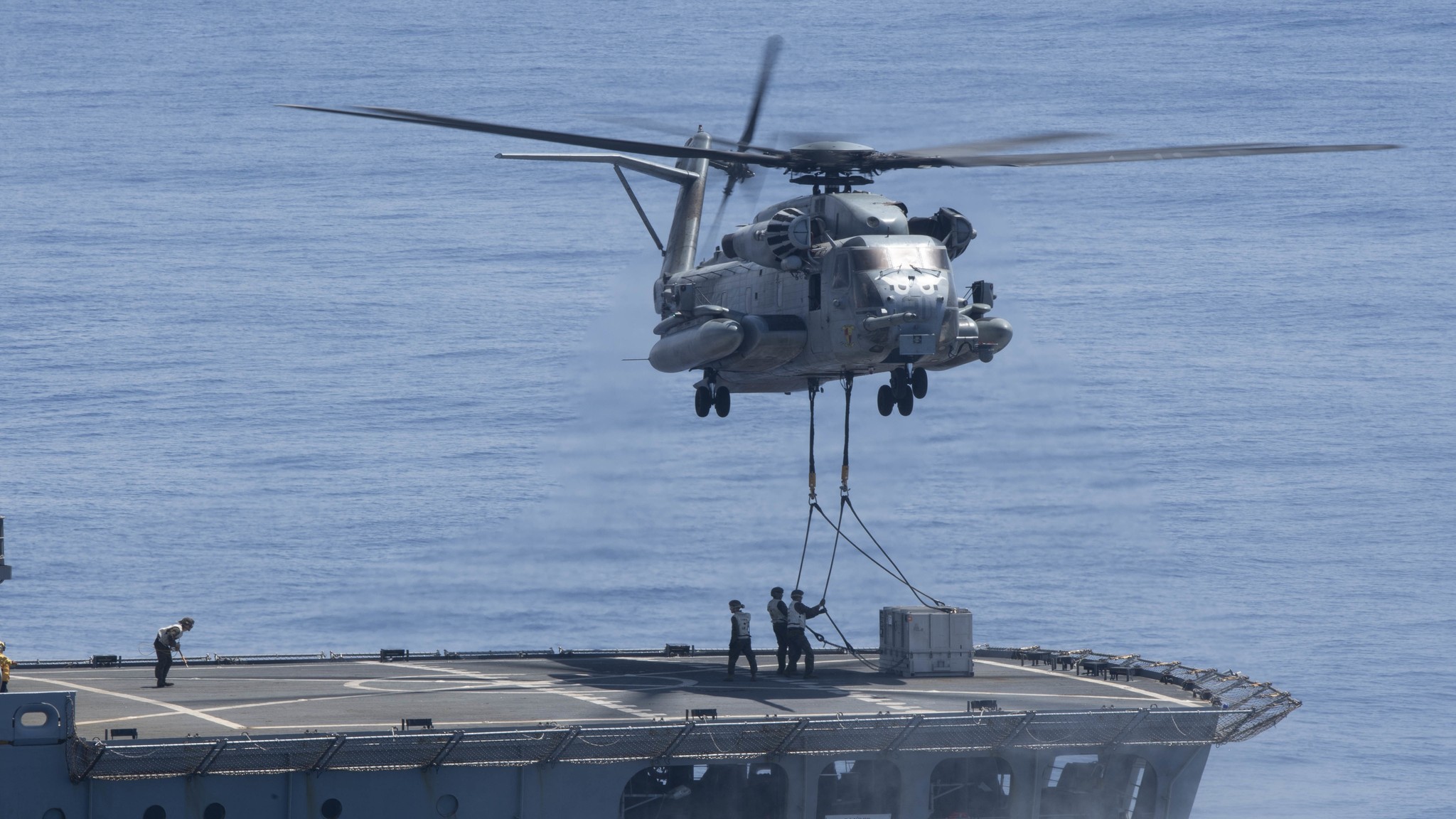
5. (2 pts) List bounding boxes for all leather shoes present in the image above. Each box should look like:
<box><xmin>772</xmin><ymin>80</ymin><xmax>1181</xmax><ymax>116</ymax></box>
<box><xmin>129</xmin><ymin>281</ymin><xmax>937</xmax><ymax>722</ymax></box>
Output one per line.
<box><xmin>157</xmin><ymin>680</ymin><xmax>174</xmax><ymax>688</ymax></box>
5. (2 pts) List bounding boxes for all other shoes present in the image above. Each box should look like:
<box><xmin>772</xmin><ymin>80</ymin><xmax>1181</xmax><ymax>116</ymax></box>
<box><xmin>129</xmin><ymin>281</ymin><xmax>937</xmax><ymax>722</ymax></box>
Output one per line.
<box><xmin>784</xmin><ymin>668</ymin><xmax>798</xmax><ymax>676</ymax></box>
<box><xmin>776</xmin><ymin>667</ymin><xmax>785</xmax><ymax>673</ymax></box>
<box><xmin>724</xmin><ymin>675</ymin><xmax>733</xmax><ymax>682</ymax></box>
<box><xmin>804</xmin><ymin>673</ymin><xmax>819</xmax><ymax>679</ymax></box>
<box><xmin>751</xmin><ymin>673</ymin><xmax>757</xmax><ymax>680</ymax></box>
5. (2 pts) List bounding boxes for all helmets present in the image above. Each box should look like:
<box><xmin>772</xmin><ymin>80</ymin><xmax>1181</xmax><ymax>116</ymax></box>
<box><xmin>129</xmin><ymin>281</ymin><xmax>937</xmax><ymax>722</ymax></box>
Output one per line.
<box><xmin>729</xmin><ymin>600</ymin><xmax>745</xmax><ymax>609</ymax></box>
<box><xmin>790</xmin><ymin>590</ymin><xmax>804</xmax><ymax>598</ymax></box>
<box><xmin>770</xmin><ymin>587</ymin><xmax>784</xmax><ymax>596</ymax></box>
<box><xmin>0</xmin><ymin>641</ymin><xmax>6</xmax><ymax>651</ymax></box>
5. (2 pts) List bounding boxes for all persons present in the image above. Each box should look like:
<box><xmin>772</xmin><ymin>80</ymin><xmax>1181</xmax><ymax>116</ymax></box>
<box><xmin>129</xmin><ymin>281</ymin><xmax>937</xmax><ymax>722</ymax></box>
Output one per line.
<box><xmin>767</xmin><ymin>587</ymin><xmax>805</xmax><ymax>675</ymax></box>
<box><xmin>717</xmin><ymin>600</ymin><xmax>759</xmax><ymax>682</ymax></box>
<box><xmin>0</xmin><ymin>641</ymin><xmax>19</xmax><ymax>668</ymax></box>
<box><xmin>156</xmin><ymin>617</ymin><xmax>195</xmax><ymax>688</ymax></box>
<box><xmin>785</xmin><ymin>590</ymin><xmax>827</xmax><ymax>678</ymax></box>
<box><xmin>1</xmin><ymin>662</ymin><xmax>10</xmax><ymax>693</ymax></box>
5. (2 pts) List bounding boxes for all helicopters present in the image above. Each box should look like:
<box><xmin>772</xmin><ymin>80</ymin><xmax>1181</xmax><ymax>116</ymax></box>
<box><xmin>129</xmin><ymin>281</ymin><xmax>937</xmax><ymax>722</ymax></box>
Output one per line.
<box><xmin>269</xmin><ymin>37</ymin><xmax>1402</xmax><ymax>420</ymax></box>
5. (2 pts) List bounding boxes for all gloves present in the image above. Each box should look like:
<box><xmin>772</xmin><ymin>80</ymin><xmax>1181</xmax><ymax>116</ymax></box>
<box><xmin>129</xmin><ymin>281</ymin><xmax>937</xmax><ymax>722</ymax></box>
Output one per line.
<box><xmin>820</xmin><ymin>608</ymin><xmax>827</xmax><ymax>614</ymax></box>
<box><xmin>820</xmin><ymin>599</ymin><xmax>826</xmax><ymax>606</ymax></box>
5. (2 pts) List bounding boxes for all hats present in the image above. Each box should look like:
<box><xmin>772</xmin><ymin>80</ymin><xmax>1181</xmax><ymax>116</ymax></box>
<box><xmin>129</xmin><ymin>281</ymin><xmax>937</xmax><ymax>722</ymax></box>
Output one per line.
<box><xmin>178</xmin><ymin>617</ymin><xmax>195</xmax><ymax>626</ymax></box>
